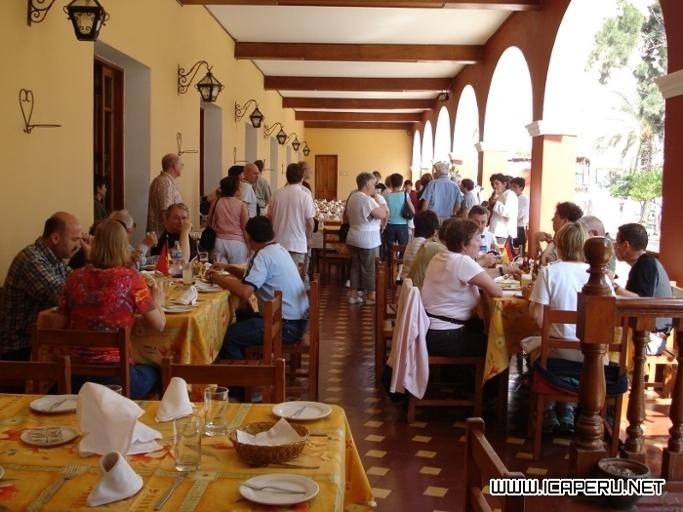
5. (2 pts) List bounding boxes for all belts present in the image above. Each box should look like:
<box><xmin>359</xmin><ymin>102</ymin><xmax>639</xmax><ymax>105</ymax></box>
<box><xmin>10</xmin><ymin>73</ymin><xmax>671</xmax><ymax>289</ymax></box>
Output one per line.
<box><xmin>654</xmin><ymin>331</ymin><xmax>667</xmax><ymax>340</ymax></box>
<box><xmin>282</xmin><ymin>319</ymin><xmax>306</xmax><ymax>323</ymax></box>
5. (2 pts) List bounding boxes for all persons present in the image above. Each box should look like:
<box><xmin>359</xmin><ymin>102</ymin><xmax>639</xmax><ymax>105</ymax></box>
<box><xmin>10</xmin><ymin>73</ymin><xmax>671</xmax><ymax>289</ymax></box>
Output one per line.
<box><xmin>68</xmin><ymin>153</ymin><xmax>618</xmax><ymax>304</ymax></box>
<box><xmin>612</xmin><ymin>223</ymin><xmax>673</xmax><ymax>357</ymax></box>
<box><xmin>528</xmin><ymin>222</ymin><xmax>616</xmax><ymax>434</ymax></box>
<box><xmin>58</xmin><ymin>218</ymin><xmax>167</xmax><ymax>400</ymax></box>
<box><xmin>204</xmin><ymin>215</ymin><xmax>310</xmax><ymax>398</ymax></box>
<box><xmin>421</xmin><ymin>219</ymin><xmax>503</xmax><ymax>409</ymax></box>
<box><xmin>0</xmin><ymin>211</ymin><xmax>95</xmax><ymax>395</ymax></box>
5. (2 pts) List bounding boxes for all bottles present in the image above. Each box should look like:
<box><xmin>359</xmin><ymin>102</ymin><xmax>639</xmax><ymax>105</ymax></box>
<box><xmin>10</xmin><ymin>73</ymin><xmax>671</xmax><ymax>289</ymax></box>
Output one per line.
<box><xmin>172</xmin><ymin>242</ymin><xmax>182</xmax><ymax>276</ymax></box>
<box><xmin>520</xmin><ymin>247</ymin><xmax>543</xmax><ymax>278</ymax></box>
<box><xmin>478</xmin><ymin>234</ymin><xmax>489</xmax><ymax>258</ymax></box>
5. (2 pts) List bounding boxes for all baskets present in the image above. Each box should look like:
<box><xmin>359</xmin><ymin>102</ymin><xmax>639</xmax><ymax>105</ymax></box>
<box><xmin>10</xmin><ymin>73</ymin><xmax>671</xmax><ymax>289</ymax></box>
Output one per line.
<box><xmin>227</xmin><ymin>422</ymin><xmax>310</xmax><ymax>464</ymax></box>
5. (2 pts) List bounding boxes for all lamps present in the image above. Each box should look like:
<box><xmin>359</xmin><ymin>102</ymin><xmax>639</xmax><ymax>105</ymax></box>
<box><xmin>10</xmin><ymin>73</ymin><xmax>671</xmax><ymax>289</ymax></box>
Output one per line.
<box><xmin>265</xmin><ymin>122</ymin><xmax>287</xmax><ymax>144</ymax></box>
<box><xmin>438</xmin><ymin>91</ymin><xmax>448</xmax><ymax>103</ymax></box>
<box><xmin>301</xmin><ymin>141</ymin><xmax>310</xmax><ymax>156</ymax></box>
<box><xmin>234</xmin><ymin>99</ymin><xmax>263</xmax><ymax>127</ymax></box>
<box><xmin>27</xmin><ymin>1</ymin><xmax>106</xmax><ymax>41</ymax></box>
<box><xmin>178</xmin><ymin>61</ymin><xmax>223</xmax><ymax>102</ymax></box>
<box><xmin>287</xmin><ymin>132</ymin><xmax>300</xmax><ymax>151</ymax></box>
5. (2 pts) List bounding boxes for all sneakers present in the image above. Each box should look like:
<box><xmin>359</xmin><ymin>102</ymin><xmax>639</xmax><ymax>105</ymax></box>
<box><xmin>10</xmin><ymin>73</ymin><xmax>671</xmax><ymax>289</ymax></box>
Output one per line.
<box><xmin>366</xmin><ymin>299</ymin><xmax>376</xmax><ymax>305</ymax></box>
<box><xmin>348</xmin><ymin>297</ymin><xmax>364</xmax><ymax>304</ymax></box>
<box><xmin>559</xmin><ymin>408</ymin><xmax>574</xmax><ymax>434</ymax></box>
<box><xmin>543</xmin><ymin>409</ymin><xmax>560</xmax><ymax>436</ymax></box>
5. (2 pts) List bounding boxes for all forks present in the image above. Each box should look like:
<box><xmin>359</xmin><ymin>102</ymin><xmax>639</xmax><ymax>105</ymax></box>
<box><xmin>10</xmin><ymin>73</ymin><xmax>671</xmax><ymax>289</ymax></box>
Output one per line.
<box><xmin>247</xmin><ymin>482</ymin><xmax>308</xmax><ymax>495</ymax></box>
<box><xmin>41</xmin><ymin>463</ymin><xmax>80</xmax><ymax>504</ymax></box>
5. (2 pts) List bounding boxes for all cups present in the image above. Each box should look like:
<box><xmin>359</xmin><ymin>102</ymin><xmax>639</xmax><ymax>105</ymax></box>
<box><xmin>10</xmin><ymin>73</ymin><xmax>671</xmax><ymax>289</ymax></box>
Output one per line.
<box><xmin>199</xmin><ymin>252</ymin><xmax>208</xmax><ymax>265</ymax></box>
<box><xmin>105</xmin><ymin>384</ymin><xmax>123</xmax><ymax>395</ymax></box>
<box><xmin>521</xmin><ymin>274</ymin><xmax>532</xmax><ymax>290</ymax></box>
<box><xmin>173</xmin><ymin>414</ymin><xmax>201</xmax><ymax>472</ymax></box>
<box><xmin>204</xmin><ymin>387</ymin><xmax>228</xmax><ymax>437</ymax></box>
<box><xmin>183</xmin><ymin>263</ymin><xmax>193</xmax><ymax>288</ymax></box>
<box><xmin>146</xmin><ymin>231</ymin><xmax>158</xmax><ymax>245</ymax></box>
<box><xmin>375</xmin><ymin>188</ymin><xmax>381</xmax><ymax>195</ymax></box>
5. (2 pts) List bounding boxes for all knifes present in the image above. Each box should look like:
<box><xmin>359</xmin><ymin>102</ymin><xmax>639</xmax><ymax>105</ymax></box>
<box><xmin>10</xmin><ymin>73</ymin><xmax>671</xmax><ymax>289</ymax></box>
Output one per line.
<box><xmin>154</xmin><ymin>474</ymin><xmax>189</xmax><ymax>511</ymax></box>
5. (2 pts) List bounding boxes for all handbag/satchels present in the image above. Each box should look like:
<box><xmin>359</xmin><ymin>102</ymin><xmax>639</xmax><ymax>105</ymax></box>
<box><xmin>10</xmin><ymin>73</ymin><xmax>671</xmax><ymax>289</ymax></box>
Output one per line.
<box><xmin>198</xmin><ymin>226</ymin><xmax>215</xmax><ymax>252</ymax></box>
<box><xmin>533</xmin><ymin>358</ymin><xmax>628</xmax><ymax>395</ymax></box>
<box><xmin>465</xmin><ymin>317</ymin><xmax>484</xmax><ymax>332</ymax></box>
<box><xmin>403</xmin><ymin>203</ymin><xmax>414</xmax><ymax>220</ymax></box>
<box><xmin>338</xmin><ymin>224</ymin><xmax>349</xmax><ymax>241</ymax></box>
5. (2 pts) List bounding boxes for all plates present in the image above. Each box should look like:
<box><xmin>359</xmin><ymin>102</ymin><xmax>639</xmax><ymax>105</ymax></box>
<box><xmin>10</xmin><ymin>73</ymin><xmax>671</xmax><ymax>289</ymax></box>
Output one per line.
<box><xmin>19</xmin><ymin>426</ymin><xmax>79</xmax><ymax>446</ymax></box>
<box><xmin>161</xmin><ymin>304</ymin><xmax>196</xmax><ymax>313</ymax></box>
<box><xmin>272</xmin><ymin>401</ymin><xmax>333</xmax><ymax>420</ymax></box>
<box><xmin>29</xmin><ymin>395</ymin><xmax>81</xmax><ymax>413</ymax></box>
<box><xmin>240</xmin><ymin>473</ymin><xmax>319</xmax><ymax>506</ymax></box>
